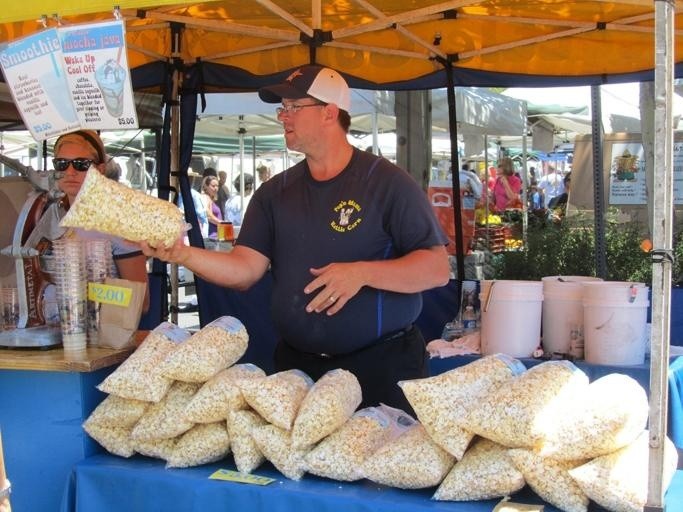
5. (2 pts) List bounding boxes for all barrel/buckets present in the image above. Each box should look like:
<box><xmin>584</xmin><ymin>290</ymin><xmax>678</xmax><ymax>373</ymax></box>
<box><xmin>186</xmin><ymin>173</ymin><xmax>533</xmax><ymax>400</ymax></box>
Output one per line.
<box><xmin>479</xmin><ymin>280</ymin><xmax>544</xmax><ymax>360</ymax></box>
<box><xmin>580</xmin><ymin>282</ymin><xmax>649</xmax><ymax>364</ymax></box>
<box><xmin>542</xmin><ymin>276</ymin><xmax>603</xmax><ymax>354</ymax></box>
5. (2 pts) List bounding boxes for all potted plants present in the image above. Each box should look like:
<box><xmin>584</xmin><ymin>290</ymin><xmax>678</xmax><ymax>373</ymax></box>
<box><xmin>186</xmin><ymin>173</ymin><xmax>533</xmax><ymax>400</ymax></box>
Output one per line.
<box><xmin>479</xmin><ymin>210</ymin><xmax>652</xmax><ymax>368</ymax></box>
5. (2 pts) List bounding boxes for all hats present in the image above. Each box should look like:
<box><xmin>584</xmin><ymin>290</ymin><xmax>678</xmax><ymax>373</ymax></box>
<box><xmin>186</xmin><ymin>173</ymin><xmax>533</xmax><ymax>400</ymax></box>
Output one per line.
<box><xmin>234</xmin><ymin>173</ymin><xmax>253</xmax><ymax>184</ymax></box>
<box><xmin>257</xmin><ymin>64</ymin><xmax>350</xmax><ymax>114</ymax></box>
<box><xmin>256</xmin><ymin>167</ymin><xmax>269</xmax><ymax>173</ymax></box>
<box><xmin>54</xmin><ymin>130</ymin><xmax>105</xmax><ymax>163</ymax></box>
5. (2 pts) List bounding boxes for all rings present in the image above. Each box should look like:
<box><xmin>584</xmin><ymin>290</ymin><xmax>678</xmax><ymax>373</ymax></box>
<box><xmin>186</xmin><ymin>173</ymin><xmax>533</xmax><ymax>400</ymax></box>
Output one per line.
<box><xmin>330</xmin><ymin>297</ymin><xmax>336</xmax><ymax>302</ymax></box>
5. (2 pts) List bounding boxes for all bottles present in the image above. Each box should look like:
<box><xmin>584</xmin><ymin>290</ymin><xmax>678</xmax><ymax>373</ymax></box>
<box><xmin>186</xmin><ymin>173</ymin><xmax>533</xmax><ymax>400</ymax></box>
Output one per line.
<box><xmin>462</xmin><ymin>306</ymin><xmax>477</xmax><ymax>336</ymax></box>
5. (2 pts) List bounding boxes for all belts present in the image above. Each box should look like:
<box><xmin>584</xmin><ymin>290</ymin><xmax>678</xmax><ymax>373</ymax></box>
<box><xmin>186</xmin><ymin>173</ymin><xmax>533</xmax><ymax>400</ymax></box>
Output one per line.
<box><xmin>302</xmin><ymin>324</ymin><xmax>413</xmax><ymax>361</ymax></box>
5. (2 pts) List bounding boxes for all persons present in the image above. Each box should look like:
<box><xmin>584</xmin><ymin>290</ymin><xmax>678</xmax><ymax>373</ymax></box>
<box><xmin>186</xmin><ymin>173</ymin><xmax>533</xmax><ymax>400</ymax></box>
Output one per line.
<box><xmin>40</xmin><ymin>130</ymin><xmax>151</xmax><ymax>316</ymax></box>
<box><xmin>174</xmin><ymin>165</ymin><xmax>271</xmax><ymax>239</ymax></box>
<box><xmin>120</xmin><ymin>65</ymin><xmax>452</xmax><ymax>420</ymax></box>
<box><xmin>461</xmin><ymin>155</ymin><xmax>571</xmax><ymax>212</ymax></box>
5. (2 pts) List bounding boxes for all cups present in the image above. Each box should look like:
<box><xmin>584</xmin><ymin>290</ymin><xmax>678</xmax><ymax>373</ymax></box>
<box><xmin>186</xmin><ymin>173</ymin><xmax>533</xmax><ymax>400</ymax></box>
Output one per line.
<box><xmin>50</xmin><ymin>238</ymin><xmax>113</xmax><ymax>351</ymax></box>
<box><xmin>95</xmin><ymin>65</ymin><xmax>128</xmax><ymax>118</ymax></box>
<box><xmin>39</xmin><ymin>68</ymin><xmax>80</xmax><ymax>123</ymax></box>
<box><xmin>0</xmin><ymin>287</ymin><xmax>19</xmax><ymax>330</ymax></box>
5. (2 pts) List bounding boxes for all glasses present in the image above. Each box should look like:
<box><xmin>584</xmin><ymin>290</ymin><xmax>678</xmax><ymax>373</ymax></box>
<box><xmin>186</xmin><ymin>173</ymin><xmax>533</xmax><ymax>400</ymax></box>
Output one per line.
<box><xmin>51</xmin><ymin>157</ymin><xmax>104</xmax><ymax>171</ymax></box>
<box><xmin>275</xmin><ymin>103</ymin><xmax>327</xmax><ymax>114</ymax></box>
